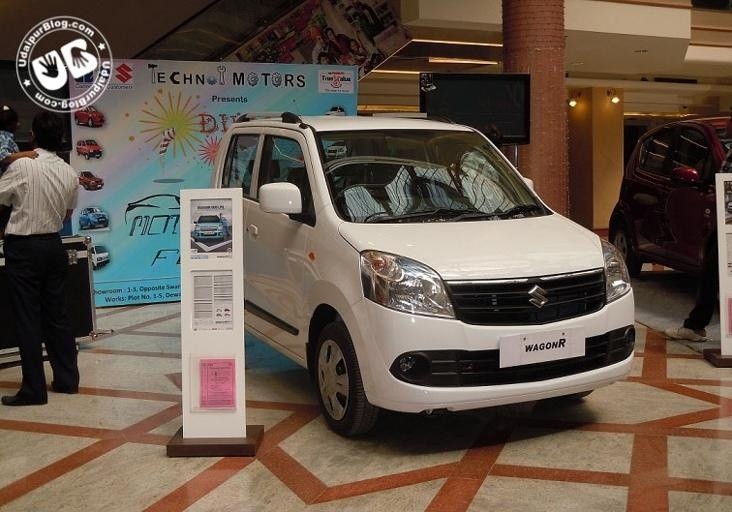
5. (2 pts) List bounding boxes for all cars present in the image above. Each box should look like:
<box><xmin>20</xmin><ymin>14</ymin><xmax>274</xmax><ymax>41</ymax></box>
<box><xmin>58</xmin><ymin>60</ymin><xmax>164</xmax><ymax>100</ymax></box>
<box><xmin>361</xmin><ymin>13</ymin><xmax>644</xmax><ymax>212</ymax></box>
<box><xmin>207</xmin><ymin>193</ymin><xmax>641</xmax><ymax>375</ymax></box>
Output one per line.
<box><xmin>91</xmin><ymin>246</ymin><xmax>109</xmax><ymax>270</ymax></box>
<box><xmin>80</xmin><ymin>172</ymin><xmax>103</xmax><ymax>190</ymax></box>
<box><xmin>213</xmin><ymin>112</ymin><xmax>636</xmax><ymax>438</ymax></box>
<box><xmin>609</xmin><ymin>116</ymin><xmax>732</xmax><ymax>278</ymax></box>
<box><xmin>194</xmin><ymin>215</ymin><xmax>225</xmax><ymax>240</ymax></box>
<box><xmin>75</xmin><ymin>106</ymin><xmax>104</xmax><ymax>128</ymax></box>
<box><xmin>76</xmin><ymin>139</ymin><xmax>103</xmax><ymax>159</ymax></box>
<box><xmin>79</xmin><ymin>207</ymin><xmax>108</xmax><ymax>229</ymax></box>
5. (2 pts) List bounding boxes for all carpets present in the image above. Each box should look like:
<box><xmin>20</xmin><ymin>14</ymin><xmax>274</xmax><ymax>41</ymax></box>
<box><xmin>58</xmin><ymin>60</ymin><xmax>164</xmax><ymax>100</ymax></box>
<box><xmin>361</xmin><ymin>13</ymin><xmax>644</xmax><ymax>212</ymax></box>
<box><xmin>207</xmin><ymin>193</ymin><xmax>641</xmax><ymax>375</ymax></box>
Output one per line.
<box><xmin>631</xmin><ymin>270</ymin><xmax>721</xmax><ymax>352</ymax></box>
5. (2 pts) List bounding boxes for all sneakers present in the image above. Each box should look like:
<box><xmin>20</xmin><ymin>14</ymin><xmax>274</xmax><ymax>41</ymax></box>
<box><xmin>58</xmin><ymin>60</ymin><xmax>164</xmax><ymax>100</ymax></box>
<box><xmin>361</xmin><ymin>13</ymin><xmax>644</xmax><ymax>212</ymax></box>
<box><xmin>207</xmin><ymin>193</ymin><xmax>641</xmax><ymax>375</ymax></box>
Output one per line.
<box><xmin>663</xmin><ymin>325</ymin><xmax>705</xmax><ymax>342</ymax></box>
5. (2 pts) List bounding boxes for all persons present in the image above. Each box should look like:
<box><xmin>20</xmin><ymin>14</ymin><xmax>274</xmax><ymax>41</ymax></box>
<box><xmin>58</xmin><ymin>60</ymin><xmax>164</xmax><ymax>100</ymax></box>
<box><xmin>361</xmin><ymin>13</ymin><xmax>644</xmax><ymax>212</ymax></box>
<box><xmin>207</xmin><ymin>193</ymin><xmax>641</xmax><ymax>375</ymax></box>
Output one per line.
<box><xmin>1</xmin><ymin>110</ymin><xmax>39</xmax><ymax>254</ymax></box>
<box><xmin>308</xmin><ymin>27</ymin><xmax>378</xmax><ymax>82</ymax></box>
<box><xmin>2</xmin><ymin>112</ymin><xmax>79</xmax><ymax>406</ymax></box>
<box><xmin>663</xmin><ymin>231</ymin><xmax>719</xmax><ymax>342</ymax></box>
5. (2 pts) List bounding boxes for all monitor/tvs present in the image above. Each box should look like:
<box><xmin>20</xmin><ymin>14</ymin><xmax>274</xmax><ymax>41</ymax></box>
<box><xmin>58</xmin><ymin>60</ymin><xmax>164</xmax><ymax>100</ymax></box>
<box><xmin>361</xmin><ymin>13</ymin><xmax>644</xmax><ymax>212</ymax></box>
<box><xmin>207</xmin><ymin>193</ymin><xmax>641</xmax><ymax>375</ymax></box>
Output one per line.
<box><xmin>418</xmin><ymin>71</ymin><xmax>532</xmax><ymax>146</ymax></box>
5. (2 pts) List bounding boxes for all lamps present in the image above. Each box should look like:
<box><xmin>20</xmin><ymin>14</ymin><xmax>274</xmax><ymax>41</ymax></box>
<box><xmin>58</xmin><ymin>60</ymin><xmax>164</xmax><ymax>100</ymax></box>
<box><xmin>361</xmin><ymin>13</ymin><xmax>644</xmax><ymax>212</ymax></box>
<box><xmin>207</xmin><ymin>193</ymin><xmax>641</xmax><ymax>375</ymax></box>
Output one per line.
<box><xmin>606</xmin><ymin>88</ymin><xmax>619</xmax><ymax>104</ymax></box>
<box><xmin>568</xmin><ymin>92</ymin><xmax>581</xmax><ymax>107</ymax></box>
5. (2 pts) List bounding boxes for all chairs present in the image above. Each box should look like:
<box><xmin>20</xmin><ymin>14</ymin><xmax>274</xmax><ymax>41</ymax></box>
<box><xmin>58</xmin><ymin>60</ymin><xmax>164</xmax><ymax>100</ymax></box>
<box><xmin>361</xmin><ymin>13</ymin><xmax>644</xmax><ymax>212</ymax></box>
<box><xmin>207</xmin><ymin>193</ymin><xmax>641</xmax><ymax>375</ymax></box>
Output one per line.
<box><xmin>247</xmin><ymin>159</ymin><xmax>311</xmax><ymax>214</ymax></box>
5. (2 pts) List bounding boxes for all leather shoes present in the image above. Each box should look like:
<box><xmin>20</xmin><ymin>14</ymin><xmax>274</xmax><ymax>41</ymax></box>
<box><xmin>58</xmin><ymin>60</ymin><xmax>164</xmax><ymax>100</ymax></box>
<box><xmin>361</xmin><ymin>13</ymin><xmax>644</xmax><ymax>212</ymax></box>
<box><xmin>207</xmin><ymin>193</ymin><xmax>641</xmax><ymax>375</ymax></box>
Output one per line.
<box><xmin>51</xmin><ymin>380</ymin><xmax>77</xmax><ymax>394</ymax></box>
<box><xmin>1</xmin><ymin>390</ymin><xmax>47</xmax><ymax>406</ymax></box>
